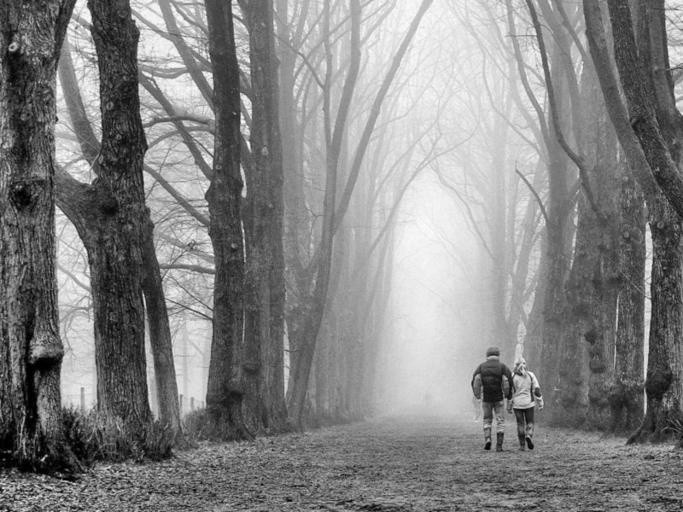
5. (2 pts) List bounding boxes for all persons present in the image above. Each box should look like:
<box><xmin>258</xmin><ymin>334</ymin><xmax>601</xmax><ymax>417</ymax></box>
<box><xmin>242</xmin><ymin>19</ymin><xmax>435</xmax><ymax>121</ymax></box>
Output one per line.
<box><xmin>506</xmin><ymin>357</ymin><xmax>543</xmax><ymax>450</ymax></box>
<box><xmin>470</xmin><ymin>346</ymin><xmax>513</xmax><ymax>450</ymax></box>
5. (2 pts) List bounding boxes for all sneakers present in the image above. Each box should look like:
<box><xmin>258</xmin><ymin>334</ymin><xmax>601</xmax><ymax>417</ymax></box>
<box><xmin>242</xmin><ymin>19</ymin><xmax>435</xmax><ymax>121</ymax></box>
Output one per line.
<box><xmin>484</xmin><ymin>440</ymin><xmax>491</xmax><ymax>450</ymax></box>
<box><xmin>519</xmin><ymin>435</ymin><xmax>534</xmax><ymax>450</ymax></box>
